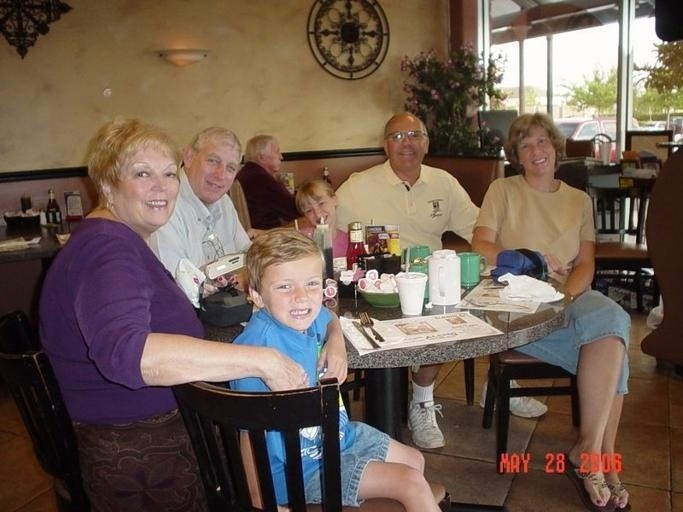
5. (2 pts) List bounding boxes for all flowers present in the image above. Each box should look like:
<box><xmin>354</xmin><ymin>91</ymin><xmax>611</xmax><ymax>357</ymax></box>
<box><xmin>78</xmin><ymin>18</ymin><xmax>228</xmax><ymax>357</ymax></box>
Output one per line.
<box><xmin>400</xmin><ymin>42</ymin><xmax>511</xmax><ymax>157</ymax></box>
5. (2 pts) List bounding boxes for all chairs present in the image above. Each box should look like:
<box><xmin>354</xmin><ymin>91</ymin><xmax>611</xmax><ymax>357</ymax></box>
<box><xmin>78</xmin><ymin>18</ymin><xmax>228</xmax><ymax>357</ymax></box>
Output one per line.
<box><xmin>463</xmin><ymin>357</ymin><xmax>477</xmax><ymax>407</ymax></box>
<box><xmin>590</xmin><ymin>186</ymin><xmax>660</xmax><ymax>314</ymax></box>
<box><xmin>623</xmin><ymin>130</ymin><xmax>674</xmax><ymax>167</ymax></box>
<box><xmin>228</xmin><ymin>181</ymin><xmax>252</xmax><ymax>234</ymax></box>
<box><xmin>338</xmin><ymin>366</ymin><xmax>369</xmax><ymax>421</ymax></box>
<box><xmin>480</xmin><ymin>347</ymin><xmax>582</xmax><ymax>475</ymax></box>
<box><xmin>172</xmin><ymin>376</ymin><xmax>343</xmax><ymax>512</ymax></box>
<box><xmin>422</xmin><ymin>159</ymin><xmax>506</xmax><ymax>254</ymax></box>
<box><xmin>565</xmin><ymin>137</ymin><xmax>596</xmax><ymax>156</ymax></box>
<box><xmin>1</xmin><ymin>312</ymin><xmax>83</xmax><ymax>512</ymax></box>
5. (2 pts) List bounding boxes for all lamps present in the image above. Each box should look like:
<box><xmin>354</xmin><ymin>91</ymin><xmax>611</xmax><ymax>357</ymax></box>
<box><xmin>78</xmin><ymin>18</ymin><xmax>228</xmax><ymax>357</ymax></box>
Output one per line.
<box><xmin>151</xmin><ymin>34</ymin><xmax>214</xmax><ymax>71</ymax></box>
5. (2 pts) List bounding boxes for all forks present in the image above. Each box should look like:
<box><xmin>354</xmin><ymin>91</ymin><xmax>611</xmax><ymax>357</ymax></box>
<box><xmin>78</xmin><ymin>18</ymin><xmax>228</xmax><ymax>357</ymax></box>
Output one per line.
<box><xmin>359</xmin><ymin>311</ymin><xmax>385</xmax><ymax>343</ymax></box>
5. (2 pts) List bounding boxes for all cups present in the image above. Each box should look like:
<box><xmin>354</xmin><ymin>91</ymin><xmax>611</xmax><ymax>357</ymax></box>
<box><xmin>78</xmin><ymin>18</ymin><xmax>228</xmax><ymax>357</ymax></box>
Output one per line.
<box><xmin>409</xmin><ymin>263</ymin><xmax>429</xmax><ymax>299</ymax></box>
<box><xmin>428</xmin><ymin>249</ymin><xmax>461</xmax><ymax>306</ymax></box>
<box><xmin>457</xmin><ymin>251</ymin><xmax>488</xmax><ymax>287</ymax></box>
<box><xmin>395</xmin><ymin>272</ymin><xmax>427</xmax><ymax>316</ymax></box>
<box><xmin>404</xmin><ymin>245</ymin><xmax>429</xmax><ymax>262</ymax></box>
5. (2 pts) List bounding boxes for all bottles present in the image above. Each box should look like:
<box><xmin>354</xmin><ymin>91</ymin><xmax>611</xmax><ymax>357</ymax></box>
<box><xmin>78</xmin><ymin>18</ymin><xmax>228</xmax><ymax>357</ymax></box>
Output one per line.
<box><xmin>22</xmin><ymin>191</ymin><xmax>32</xmax><ymax>214</ymax></box>
<box><xmin>321</xmin><ymin>168</ymin><xmax>331</xmax><ymax>187</ymax></box>
<box><xmin>45</xmin><ymin>189</ymin><xmax>62</xmax><ymax>225</ymax></box>
<box><xmin>311</xmin><ymin>217</ymin><xmax>334</xmax><ymax>288</ymax></box>
<box><xmin>39</xmin><ymin>209</ymin><xmax>50</xmax><ymax>240</ymax></box>
<box><xmin>346</xmin><ymin>222</ymin><xmax>368</xmax><ymax>269</ymax></box>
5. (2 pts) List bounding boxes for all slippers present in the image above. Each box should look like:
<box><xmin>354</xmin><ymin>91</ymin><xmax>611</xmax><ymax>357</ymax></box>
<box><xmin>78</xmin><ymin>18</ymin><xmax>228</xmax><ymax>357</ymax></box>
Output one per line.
<box><xmin>607</xmin><ymin>480</ymin><xmax>631</xmax><ymax>512</ymax></box>
<box><xmin>562</xmin><ymin>453</ymin><xmax>615</xmax><ymax>512</ymax></box>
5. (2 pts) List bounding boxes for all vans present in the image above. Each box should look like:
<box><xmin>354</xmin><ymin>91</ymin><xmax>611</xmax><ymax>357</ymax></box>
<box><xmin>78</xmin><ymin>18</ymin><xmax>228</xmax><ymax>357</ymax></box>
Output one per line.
<box><xmin>550</xmin><ymin>117</ymin><xmax>641</xmax><ymax>163</ymax></box>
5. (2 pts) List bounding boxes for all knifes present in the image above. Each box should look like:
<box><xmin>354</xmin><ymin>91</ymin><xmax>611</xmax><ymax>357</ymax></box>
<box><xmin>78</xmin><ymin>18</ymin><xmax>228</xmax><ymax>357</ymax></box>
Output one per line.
<box><xmin>351</xmin><ymin>320</ymin><xmax>380</xmax><ymax>350</ymax></box>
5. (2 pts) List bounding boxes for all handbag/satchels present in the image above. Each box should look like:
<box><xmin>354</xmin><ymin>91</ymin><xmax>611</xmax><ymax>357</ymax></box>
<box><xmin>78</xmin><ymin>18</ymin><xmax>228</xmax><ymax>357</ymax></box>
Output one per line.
<box><xmin>490</xmin><ymin>247</ymin><xmax>550</xmax><ymax>279</ymax></box>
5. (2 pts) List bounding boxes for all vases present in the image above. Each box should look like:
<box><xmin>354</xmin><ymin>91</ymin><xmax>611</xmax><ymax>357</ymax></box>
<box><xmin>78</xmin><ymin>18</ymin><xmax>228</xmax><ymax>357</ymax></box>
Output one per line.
<box><xmin>426</xmin><ymin>141</ymin><xmax>458</xmax><ymax>156</ymax></box>
<box><xmin>462</xmin><ymin>148</ymin><xmax>502</xmax><ymax>157</ymax></box>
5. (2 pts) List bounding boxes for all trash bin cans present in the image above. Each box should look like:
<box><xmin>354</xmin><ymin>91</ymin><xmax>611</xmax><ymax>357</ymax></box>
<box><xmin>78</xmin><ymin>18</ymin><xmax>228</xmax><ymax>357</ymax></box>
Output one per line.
<box><xmin>646</xmin><ymin>306</ymin><xmax>664</xmax><ymax>329</ymax></box>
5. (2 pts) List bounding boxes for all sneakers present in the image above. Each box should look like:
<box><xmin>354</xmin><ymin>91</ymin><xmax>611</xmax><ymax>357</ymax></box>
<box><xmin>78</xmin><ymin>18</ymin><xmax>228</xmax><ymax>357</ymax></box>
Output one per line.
<box><xmin>479</xmin><ymin>378</ymin><xmax>548</xmax><ymax>417</ymax></box>
<box><xmin>407</xmin><ymin>400</ymin><xmax>445</xmax><ymax>448</ymax></box>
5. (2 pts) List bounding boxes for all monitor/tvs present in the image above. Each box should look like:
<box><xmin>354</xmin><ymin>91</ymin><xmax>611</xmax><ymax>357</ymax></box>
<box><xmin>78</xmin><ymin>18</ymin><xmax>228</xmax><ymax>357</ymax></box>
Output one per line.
<box><xmin>478</xmin><ymin>110</ymin><xmax>518</xmax><ymax>148</ymax></box>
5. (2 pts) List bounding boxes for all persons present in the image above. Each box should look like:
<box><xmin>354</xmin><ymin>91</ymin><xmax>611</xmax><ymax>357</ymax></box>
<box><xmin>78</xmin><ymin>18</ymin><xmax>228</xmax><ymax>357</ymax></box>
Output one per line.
<box><xmin>233</xmin><ymin>135</ymin><xmax>305</xmax><ymax>229</ymax></box>
<box><xmin>247</xmin><ymin>181</ymin><xmax>350</xmax><ymax>260</ymax></box>
<box><xmin>144</xmin><ymin>127</ymin><xmax>266</xmax><ymax>308</ymax></box>
<box><xmin>471</xmin><ymin>114</ymin><xmax>632</xmax><ymax>510</ymax></box>
<box><xmin>228</xmin><ymin>231</ymin><xmax>443</xmax><ymax>511</ymax></box>
<box><xmin>39</xmin><ymin>119</ymin><xmax>308</xmax><ymax>505</ymax></box>
<box><xmin>334</xmin><ymin>113</ymin><xmax>548</xmax><ymax>450</ymax></box>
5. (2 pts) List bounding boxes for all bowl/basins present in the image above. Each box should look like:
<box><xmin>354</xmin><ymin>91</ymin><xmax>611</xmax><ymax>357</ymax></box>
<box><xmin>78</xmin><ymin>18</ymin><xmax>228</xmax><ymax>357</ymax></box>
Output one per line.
<box><xmin>356</xmin><ymin>286</ymin><xmax>400</xmax><ymax>308</ymax></box>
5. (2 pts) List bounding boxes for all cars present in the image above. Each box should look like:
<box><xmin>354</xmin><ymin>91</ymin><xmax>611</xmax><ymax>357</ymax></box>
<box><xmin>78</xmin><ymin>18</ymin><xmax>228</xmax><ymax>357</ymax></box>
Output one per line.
<box><xmin>644</xmin><ymin>120</ymin><xmax>668</xmax><ymax>131</ymax></box>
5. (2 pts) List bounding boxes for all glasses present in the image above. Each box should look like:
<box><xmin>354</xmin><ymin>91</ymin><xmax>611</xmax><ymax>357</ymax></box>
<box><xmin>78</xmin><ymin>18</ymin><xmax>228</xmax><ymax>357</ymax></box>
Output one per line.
<box><xmin>384</xmin><ymin>130</ymin><xmax>429</xmax><ymax>141</ymax></box>
